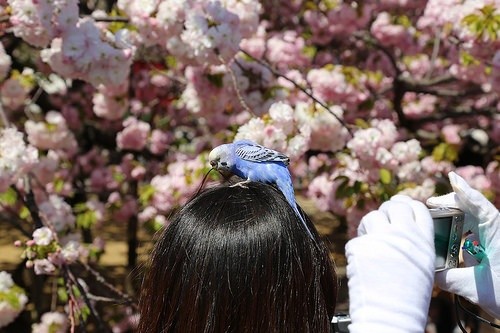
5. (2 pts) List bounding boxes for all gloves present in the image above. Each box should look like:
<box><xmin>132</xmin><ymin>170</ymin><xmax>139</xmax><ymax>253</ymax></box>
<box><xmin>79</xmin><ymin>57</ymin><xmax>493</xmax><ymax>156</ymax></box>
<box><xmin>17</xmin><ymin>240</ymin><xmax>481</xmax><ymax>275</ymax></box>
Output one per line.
<box><xmin>345</xmin><ymin>195</ymin><xmax>434</xmax><ymax>333</ymax></box>
<box><xmin>426</xmin><ymin>172</ymin><xmax>500</xmax><ymax>318</ymax></box>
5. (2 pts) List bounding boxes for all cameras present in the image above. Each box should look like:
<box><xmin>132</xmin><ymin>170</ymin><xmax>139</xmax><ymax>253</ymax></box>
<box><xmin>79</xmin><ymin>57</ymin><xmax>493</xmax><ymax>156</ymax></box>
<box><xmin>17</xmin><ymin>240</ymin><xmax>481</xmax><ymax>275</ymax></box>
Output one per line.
<box><xmin>428</xmin><ymin>208</ymin><xmax>465</xmax><ymax>272</ymax></box>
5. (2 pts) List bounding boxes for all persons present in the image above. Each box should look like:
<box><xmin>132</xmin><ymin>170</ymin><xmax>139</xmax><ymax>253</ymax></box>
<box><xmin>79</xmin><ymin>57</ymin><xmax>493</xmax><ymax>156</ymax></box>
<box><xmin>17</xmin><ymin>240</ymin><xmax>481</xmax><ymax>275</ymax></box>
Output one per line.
<box><xmin>345</xmin><ymin>172</ymin><xmax>500</xmax><ymax>333</ymax></box>
<box><xmin>137</xmin><ymin>181</ymin><xmax>339</xmax><ymax>333</ymax></box>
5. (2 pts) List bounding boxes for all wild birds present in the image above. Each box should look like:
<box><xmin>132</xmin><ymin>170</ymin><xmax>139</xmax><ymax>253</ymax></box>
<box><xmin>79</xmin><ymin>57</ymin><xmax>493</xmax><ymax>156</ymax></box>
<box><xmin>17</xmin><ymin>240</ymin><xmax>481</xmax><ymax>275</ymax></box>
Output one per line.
<box><xmin>209</xmin><ymin>138</ymin><xmax>321</xmax><ymax>252</ymax></box>
